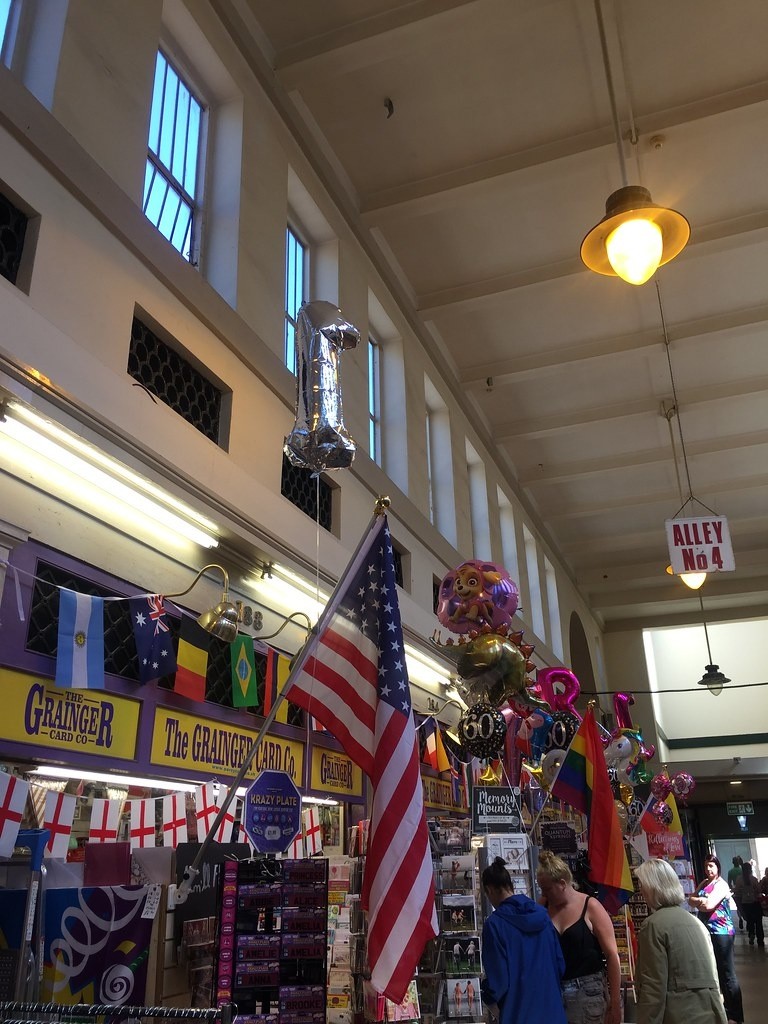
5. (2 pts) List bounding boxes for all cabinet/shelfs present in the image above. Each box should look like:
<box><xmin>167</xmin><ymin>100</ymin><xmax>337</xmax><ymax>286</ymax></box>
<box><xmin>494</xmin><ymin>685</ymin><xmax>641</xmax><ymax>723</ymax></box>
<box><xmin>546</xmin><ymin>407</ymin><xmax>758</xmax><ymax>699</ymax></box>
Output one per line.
<box><xmin>628</xmin><ymin>865</ymin><xmax>653</xmax><ymax>943</ymax></box>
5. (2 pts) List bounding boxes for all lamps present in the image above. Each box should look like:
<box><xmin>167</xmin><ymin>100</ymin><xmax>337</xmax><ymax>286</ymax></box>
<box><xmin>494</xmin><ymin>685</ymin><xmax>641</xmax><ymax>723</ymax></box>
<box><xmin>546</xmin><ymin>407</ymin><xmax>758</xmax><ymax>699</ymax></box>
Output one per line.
<box><xmin>252</xmin><ymin>612</ymin><xmax>313</xmax><ymax>672</ymax></box>
<box><xmin>697</xmin><ymin>586</ymin><xmax>732</xmax><ymax>696</ymax></box>
<box><xmin>577</xmin><ymin>0</ymin><xmax>692</xmax><ymax>286</ymax></box>
<box><xmin>658</xmin><ymin>397</ymin><xmax>706</xmax><ymax>590</ymax></box>
<box><xmin>162</xmin><ymin>564</ymin><xmax>240</xmax><ymax>643</ymax></box>
<box><xmin>418</xmin><ymin>699</ymin><xmax>464</xmax><ymax>748</ymax></box>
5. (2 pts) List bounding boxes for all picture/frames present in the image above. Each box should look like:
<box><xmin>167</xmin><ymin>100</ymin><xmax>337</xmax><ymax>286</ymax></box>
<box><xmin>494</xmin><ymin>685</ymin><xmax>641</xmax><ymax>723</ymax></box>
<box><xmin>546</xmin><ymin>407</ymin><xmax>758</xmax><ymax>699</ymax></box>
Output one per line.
<box><xmin>484</xmin><ymin>833</ymin><xmax>530</xmax><ymax>870</ymax></box>
<box><xmin>510</xmin><ymin>873</ymin><xmax>532</xmax><ymax>899</ymax></box>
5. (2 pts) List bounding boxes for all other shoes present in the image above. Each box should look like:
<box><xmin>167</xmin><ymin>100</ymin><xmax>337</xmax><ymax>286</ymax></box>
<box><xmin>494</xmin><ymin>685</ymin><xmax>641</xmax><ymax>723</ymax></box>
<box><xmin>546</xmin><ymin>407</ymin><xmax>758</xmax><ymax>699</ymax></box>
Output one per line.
<box><xmin>758</xmin><ymin>942</ymin><xmax>764</xmax><ymax>947</ymax></box>
<box><xmin>739</xmin><ymin>919</ymin><xmax>743</xmax><ymax>929</ymax></box>
<box><xmin>749</xmin><ymin>933</ymin><xmax>755</xmax><ymax>945</ymax></box>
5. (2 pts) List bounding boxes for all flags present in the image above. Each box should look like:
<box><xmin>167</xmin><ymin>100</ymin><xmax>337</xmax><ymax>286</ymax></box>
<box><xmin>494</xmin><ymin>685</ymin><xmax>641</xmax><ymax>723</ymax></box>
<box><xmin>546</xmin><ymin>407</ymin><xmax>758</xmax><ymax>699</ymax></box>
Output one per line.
<box><xmin>130</xmin><ymin>596</ymin><xmax>178</xmax><ymax>687</ymax></box>
<box><xmin>638</xmin><ymin>771</ymin><xmax>700</xmax><ymax>912</ymax></box>
<box><xmin>54</xmin><ymin>589</ymin><xmax>105</xmax><ymax>690</ymax></box>
<box><xmin>285</xmin><ymin>516</ymin><xmax>439</xmax><ymax>1005</ymax></box>
<box><xmin>173</xmin><ymin>613</ymin><xmax>211</xmax><ymax>703</ymax></box>
<box><xmin>230</xmin><ymin>634</ymin><xmax>259</xmax><ymax>707</ymax></box>
<box><xmin>0</xmin><ymin>772</ymin><xmax>322</xmax><ymax>858</ymax></box>
<box><xmin>422</xmin><ymin>716</ymin><xmax>545</xmax><ymax>813</ymax></box>
<box><xmin>550</xmin><ymin>708</ymin><xmax>634</xmax><ymax>918</ymax></box>
<box><xmin>264</xmin><ymin>648</ymin><xmax>292</xmax><ymax>724</ymax></box>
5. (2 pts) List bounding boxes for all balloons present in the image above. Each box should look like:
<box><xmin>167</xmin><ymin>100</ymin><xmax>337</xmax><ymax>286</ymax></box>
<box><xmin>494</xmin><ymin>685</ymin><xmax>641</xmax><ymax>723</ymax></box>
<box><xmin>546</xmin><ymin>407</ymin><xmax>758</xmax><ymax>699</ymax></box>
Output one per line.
<box><xmin>281</xmin><ymin>300</ymin><xmax>360</xmax><ymax>469</ymax></box>
<box><xmin>439</xmin><ymin>559</ymin><xmax>696</xmax><ymax>826</ymax></box>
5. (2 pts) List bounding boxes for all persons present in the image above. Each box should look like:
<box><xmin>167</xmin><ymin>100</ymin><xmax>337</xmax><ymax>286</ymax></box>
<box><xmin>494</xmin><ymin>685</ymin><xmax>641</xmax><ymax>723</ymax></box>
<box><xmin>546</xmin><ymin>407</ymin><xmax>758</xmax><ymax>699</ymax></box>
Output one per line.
<box><xmin>471</xmin><ymin>856</ymin><xmax>566</xmax><ymax>1024</ymax></box>
<box><xmin>506</xmin><ymin>848</ymin><xmax>524</xmax><ymax>864</ymax></box>
<box><xmin>728</xmin><ymin>854</ymin><xmax>768</xmax><ymax>947</ymax></box>
<box><xmin>450</xmin><ymin>821</ymin><xmax>463</xmax><ymax>837</ymax></box>
<box><xmin>438</xmin><ymin>860</ymin><xmax>475</xmax><ymax>1014</ymax></box>
<box><xmin>688</xmin><ymin>855</ymin><xmax>745</xmax><ymax>1024</ymax></box>
<box><xmin>634</xmin><ymin>859</ymin><xmax>728</xmax><ymax>1024</ymax></box>
<box><xmin>536</xmin><ymin>850</ymin><xmax>621</xmax><ymax>1024</ymax></box>
